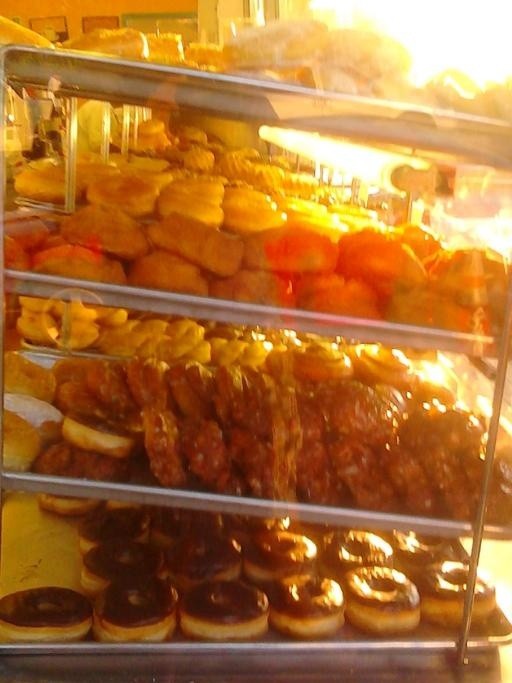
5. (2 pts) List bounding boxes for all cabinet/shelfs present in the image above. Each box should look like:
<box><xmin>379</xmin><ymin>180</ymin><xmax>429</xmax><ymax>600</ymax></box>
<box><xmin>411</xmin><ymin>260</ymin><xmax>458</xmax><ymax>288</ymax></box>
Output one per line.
<box><xmin>1</xmin><ymin>40</ymin><xmax>512</xmax><ymax>679</ymax></box>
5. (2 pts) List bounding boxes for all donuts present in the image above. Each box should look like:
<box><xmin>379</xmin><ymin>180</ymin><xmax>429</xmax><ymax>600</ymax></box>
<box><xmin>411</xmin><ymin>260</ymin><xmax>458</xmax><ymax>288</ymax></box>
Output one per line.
<box><xmin>0</xmin><ymin>492</ymin><xmax>497</xmax><ymax>645</ymax></box>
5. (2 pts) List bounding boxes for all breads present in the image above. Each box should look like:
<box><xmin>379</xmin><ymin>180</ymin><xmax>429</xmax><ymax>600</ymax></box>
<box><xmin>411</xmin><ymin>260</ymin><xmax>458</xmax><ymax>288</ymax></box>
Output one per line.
<box><xmin>0</xmin><ymin>22</ymin><xmax>512</xmax><ymax>526</ymax></box>
